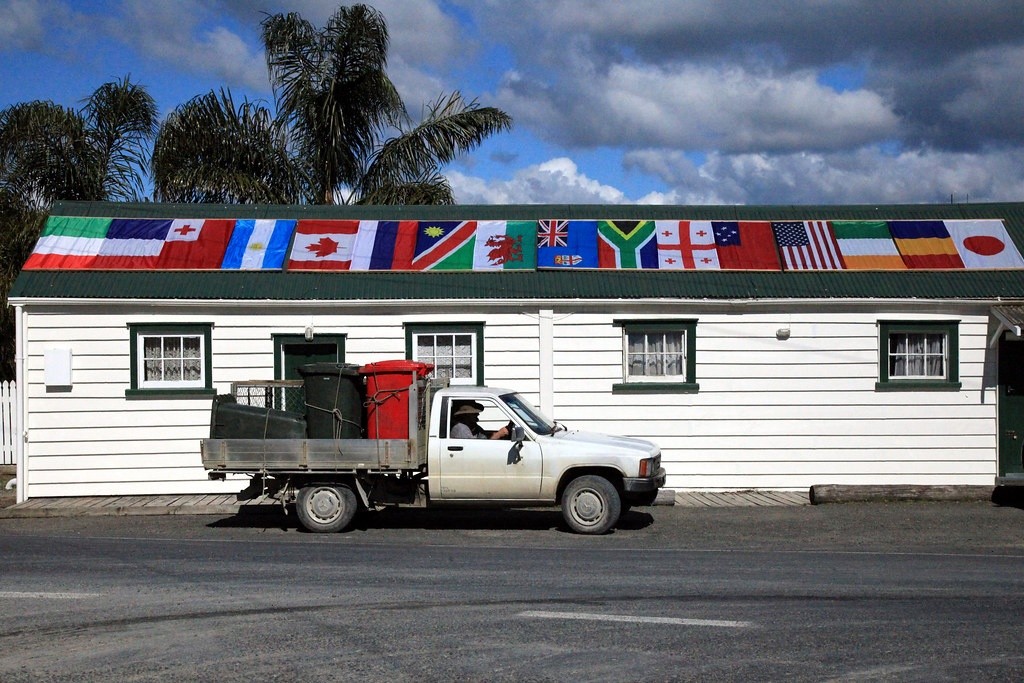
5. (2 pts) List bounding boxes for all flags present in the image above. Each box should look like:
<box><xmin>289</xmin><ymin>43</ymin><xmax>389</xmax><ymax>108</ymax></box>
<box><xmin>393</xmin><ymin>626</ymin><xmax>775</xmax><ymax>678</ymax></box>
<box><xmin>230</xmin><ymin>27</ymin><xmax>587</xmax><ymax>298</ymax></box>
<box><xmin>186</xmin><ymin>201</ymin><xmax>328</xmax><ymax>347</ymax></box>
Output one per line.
<box><xmin>23</xmin><ymin>215</ymin><xmax>1024</xmax><ymax>273</ymax></box>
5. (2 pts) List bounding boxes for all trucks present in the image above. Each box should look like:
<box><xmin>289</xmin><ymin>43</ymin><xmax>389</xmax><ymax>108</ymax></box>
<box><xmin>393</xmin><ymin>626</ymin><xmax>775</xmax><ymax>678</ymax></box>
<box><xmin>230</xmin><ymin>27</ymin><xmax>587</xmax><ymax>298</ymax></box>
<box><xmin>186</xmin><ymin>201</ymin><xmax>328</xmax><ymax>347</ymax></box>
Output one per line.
<box><xmin>199</xmin><ymin>367</ymin><xmax>667</xmax><ymax>534</ymax></box>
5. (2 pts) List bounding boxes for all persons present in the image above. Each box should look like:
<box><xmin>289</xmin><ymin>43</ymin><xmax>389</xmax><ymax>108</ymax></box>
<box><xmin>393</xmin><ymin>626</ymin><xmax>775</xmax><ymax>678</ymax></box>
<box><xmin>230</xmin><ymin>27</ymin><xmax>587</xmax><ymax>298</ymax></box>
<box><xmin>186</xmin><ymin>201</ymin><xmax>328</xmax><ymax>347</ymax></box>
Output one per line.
<box><xmin>451</xmin><ymin>403</ymin><xmax>509</xmax><ymax>440</ymax></box>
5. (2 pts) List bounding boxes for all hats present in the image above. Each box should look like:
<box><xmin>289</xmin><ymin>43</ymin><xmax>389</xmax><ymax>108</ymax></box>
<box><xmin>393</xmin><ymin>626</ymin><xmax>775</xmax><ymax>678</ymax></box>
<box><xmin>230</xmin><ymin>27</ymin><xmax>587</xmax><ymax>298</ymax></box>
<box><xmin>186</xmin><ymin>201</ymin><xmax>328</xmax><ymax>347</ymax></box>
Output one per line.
<box><xmin>452</xmin><ymin>399</ymin><xmax>484</xmax><ymax>416</ymax></box>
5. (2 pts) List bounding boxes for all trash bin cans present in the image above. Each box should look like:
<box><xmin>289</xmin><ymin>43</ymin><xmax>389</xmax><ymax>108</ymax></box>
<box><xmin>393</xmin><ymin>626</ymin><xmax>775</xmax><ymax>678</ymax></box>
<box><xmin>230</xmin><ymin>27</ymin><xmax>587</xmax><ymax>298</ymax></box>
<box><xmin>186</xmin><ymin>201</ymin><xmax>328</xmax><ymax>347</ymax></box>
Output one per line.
<box><xmin>299</xmin><ymin>362</ymin><xmax>366</xmax><ymax>439</ymax></box>
<box><xmin>208</xmin><ymin>394</ymin><xmax>308</xmax><ymax>439</ymax></box>
<box><xmin>358</xmin><ymin>359</ymin><xmax>434</xmax><ymax>442</ymax></box>
